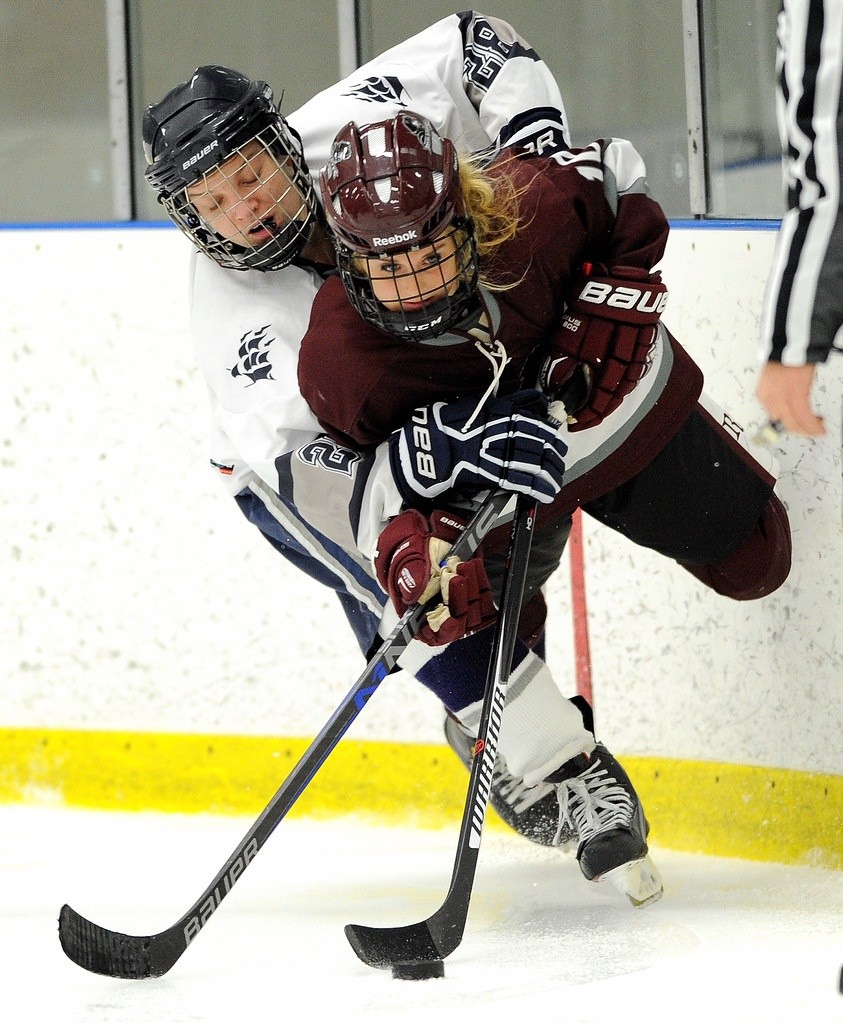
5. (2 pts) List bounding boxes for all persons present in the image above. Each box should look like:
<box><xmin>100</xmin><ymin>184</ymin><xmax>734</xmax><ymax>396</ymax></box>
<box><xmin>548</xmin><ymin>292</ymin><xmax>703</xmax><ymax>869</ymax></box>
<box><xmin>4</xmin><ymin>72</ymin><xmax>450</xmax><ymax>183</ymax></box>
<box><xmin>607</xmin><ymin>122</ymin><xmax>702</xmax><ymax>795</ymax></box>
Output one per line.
<box><xmin>296</xmin><ymin>110</ymin><xmax>795</xmax><ymax>900</ymax></box>
<box><xmin>756</xmin><ymin>0</ymin><xmax>843</xmax><ymax>436</ymax></box>
<box><xmin>140</xmin><ymin>10</ymin><xmax>573</xmax><ymax>848</ymax></box>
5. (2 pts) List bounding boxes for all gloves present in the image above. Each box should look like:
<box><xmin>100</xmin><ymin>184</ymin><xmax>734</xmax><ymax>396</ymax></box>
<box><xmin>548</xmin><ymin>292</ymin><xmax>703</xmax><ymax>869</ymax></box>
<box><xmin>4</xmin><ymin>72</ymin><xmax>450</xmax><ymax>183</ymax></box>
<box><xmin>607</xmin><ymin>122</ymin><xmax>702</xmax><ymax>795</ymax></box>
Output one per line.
<box><xmin>374</xmin><ymin>509</ymin><xmax>496</xmax><ymax>646</ymax></box>
<box><xmin>539</xmin><ymin>262</ymin><xmax>670</xmax><ymax>433</ymax></box>
<box><xmin>386</xmin><ymin>389</ymin><xmax>569</xmax><ymax>505</ymax></box>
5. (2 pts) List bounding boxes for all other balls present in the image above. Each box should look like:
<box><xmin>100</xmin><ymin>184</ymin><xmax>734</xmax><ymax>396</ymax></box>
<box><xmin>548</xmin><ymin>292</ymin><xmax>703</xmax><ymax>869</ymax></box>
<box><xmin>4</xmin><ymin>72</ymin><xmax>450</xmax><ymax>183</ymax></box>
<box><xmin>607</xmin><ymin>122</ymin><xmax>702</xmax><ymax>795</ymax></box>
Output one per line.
<box><xmin>391</xmin><ymin>959</ymin><xmax>447</xmax><ymax>981</ymax></box>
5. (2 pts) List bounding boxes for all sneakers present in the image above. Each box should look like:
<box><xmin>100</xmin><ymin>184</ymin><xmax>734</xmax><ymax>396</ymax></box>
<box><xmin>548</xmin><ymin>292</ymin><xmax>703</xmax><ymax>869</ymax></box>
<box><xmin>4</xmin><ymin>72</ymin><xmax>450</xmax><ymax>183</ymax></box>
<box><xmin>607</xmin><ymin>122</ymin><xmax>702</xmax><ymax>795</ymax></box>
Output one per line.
<box><xmin>542</xmin><ymin>741</ymin><xmax>665</xmax><ymax>911</ymax></box>
<box><xmin>444</xmin><ymin>715</ymin><xmax>579</xmax><ymax>854</ymax></box>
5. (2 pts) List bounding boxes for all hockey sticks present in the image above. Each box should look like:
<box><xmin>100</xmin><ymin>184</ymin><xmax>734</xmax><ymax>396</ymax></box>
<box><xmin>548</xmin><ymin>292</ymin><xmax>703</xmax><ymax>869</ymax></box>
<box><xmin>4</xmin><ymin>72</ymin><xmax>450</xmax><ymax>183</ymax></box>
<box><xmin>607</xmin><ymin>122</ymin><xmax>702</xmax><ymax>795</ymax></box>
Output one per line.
<box><xmin>341</xmin><ymin>377</ymin><xmax>555</xmax><ymax>971</ymax></box>
<box><xmin>56</xmin><ymin>486</ymin><xmax>514</xmax><ymax>982</ymax></box>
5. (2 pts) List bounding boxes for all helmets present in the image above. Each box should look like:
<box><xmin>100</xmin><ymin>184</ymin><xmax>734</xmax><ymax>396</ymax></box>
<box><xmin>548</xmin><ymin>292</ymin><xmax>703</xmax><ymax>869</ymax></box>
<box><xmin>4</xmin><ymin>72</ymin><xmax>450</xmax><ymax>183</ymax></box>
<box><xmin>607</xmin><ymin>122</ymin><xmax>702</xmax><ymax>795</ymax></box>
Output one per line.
<box><xmin>319</xmin><ymin>109</ymin><xmax>481</xmax><ymax>343</ymax></box>
<box><xmin>142</xmin><ymin>64</ymin><xmax>317</xmax><ymax>274</ymax></box>
